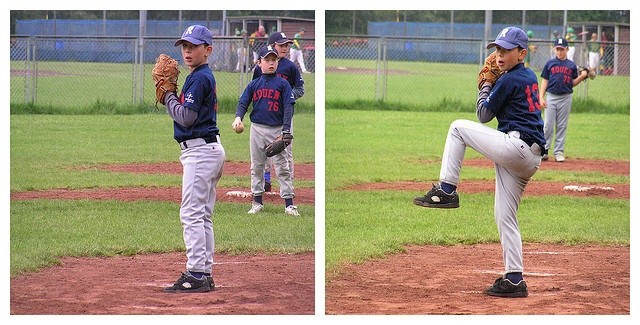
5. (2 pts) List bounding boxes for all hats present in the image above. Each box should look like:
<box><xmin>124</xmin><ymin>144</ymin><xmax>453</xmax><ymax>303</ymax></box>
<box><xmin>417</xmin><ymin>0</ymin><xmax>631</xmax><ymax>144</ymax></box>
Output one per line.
<box><xmin>175</xmin><ymin>26</ymin><xmax>212</xmax><ymax>47</ymax></box>
<box><xmin>270</xmin><ymin>31</ymin><xmax>294</xmax><ymax>46</ymax></box>
<box><xmin>300</xmin><ymin>27</ymin><xmax>308</xmax><ymax>32</ymax></box>
<box><xmin>487</xmin><ymin>27</ymin><xmax>530</xmax><ymax>49</ymax></box>
<box><xmin>259</xmin><ymin>46</ymin><xmax>278</xmax><ymax>58</ymax></box>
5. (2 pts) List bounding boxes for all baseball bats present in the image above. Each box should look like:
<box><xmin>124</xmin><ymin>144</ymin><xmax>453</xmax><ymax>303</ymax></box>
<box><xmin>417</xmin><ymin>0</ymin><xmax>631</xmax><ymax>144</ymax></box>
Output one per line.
<box><xmin>576</xmin><ymin>31</ymin><xmax>588</xmax><ymax>36</ymax></box>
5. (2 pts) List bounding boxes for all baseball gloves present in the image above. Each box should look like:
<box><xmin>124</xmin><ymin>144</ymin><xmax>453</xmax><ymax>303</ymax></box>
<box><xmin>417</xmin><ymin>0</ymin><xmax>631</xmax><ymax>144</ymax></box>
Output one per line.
<box><xmin>152</xmin><ymin>54</ymin><xmax>180</xmax><ymax>105</ymax></box>
<box><xmin>266</xmin><ymin>135</ymin><xmax>294</xmax><ymax>157</ymax></box>
<box><xmin>477</xmin><ymin>51</ymin><xmax>505</xmax><ymax>87</ymax></box>
<box><xmin>580</xmin><ymin>65</ymin><xmax>592</xmax><ymax>80</ymax></box>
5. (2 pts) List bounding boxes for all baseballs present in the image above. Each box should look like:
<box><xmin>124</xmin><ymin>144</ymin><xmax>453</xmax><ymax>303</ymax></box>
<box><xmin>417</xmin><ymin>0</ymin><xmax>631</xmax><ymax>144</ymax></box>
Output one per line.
<box><xmin>235</xmin><ymin>124</ymin><xmax>244</xmax><ymax>134</ymax></box>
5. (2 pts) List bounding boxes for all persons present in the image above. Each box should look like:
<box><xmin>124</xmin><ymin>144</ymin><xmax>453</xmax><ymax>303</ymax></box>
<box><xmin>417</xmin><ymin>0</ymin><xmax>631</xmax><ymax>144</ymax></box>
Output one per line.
<box><xmin>523</xmin><ymin>30</ymin><xmax>538</xmax><ymax>67</ymax></box>
<box><xmin>231</xmin><ymin>29</ymin><xmax>240</xmax><ymax>71</ymax></box>
<box><xmin>232</xmin><ymin>46</ymin><xmax>301</xmax><ymax>217</ymax></box>
<box><xmin>586</xmin><ymin>33</ymin><xmax>603</xmax><ymax>73</ymax></box>
<box><xmin>540</xmin><ymin>39</ymin><xmax>596</xmax><ymax>162</ymax></box>
<box><xmin>565</xmin><ymin>27</ymin><xmax>577</xmax><ymax>62</ymax></box>
<box><xmin>152</xmin><ymin>25</ymin><xmax>225</xmax><ymax>293</ymax></box>
<box><xmin>252</xmin><ymin>32</ymin><xmax>304</xmax><ymax>192</ymax></box>
<box><xmin>289</xmin><ymin>27</ymin><xmax>310</xmax><ymax>73</ymax></box>
<box><xmin>249</xmin><ymin>26</ymin><xmax>269</xmax><ymax>72</ymax></box>
<box><xmin>235</xmin><ymin>29</ymin><xmax>248</xmax><ymax>72</ymax></box>
<box><xmin>549</xmin><ymin>30</ymin><xmax>560</xmax><ymax>59</ymax></box>
<box><xmin>412</xmin><ymin>27</ymin><xmax>546</xmax><ymax>298</ymax></box>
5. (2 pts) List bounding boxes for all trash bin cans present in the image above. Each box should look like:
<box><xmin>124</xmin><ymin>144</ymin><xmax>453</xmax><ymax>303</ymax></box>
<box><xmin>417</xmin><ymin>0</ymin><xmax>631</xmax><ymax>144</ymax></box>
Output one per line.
<box><xmin>305</xmin><ymin>46</ymin><xmax>315</xmax><ymax>72</ymax></box>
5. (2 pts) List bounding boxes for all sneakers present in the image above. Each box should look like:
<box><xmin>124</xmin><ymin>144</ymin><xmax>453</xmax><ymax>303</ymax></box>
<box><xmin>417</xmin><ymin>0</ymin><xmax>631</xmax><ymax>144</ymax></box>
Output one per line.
<box><xmin>485</xmin><ymin>279</ymin><xmax>528</xmax><ymax>296</ymax></box>
<box><xmin>305</xmin><ymin>71</ymin><xmax>312</xmax><ymax>74</ymax></box>
<box><xmin>284</xmin><ymin>206</ymin><xmax>299</xmax><ymax>216</ymax></box>
<box><xmin>167</xmin><ymin>273</ymin><xmax>210</xmax><ymax>292</ymax></box>
<box><xmin>543</xmin><ymin>152</ymin><xmax>549</xmax><ymax>160</ymax></box>
<box><xmin>557</xmin><ymin>152</ymin><xmax>564</xmax><ymax>162</ymax></box>
<box><xmin>246</xmin><ymin>205</ymin><xmax>263</xmax><ymax>213</ymax></box>
<box><xmin>415</xmin><ymin>187</ymin><xmax>459</xmax><ymax>207</ymax></box>
<box><xmin>205</xmin><ymin>276</ymin><xmax>215</xmax><ymax>288</ymax></box>
<box><xmin>553</xmin><ymin>36</ymin><xmax>569</xmax><ymax>49</ymax></box>
<box><xmin>265</xmin><ymin>183</ymin><xmax>272</xmax><ymax>189</ymax></box>
